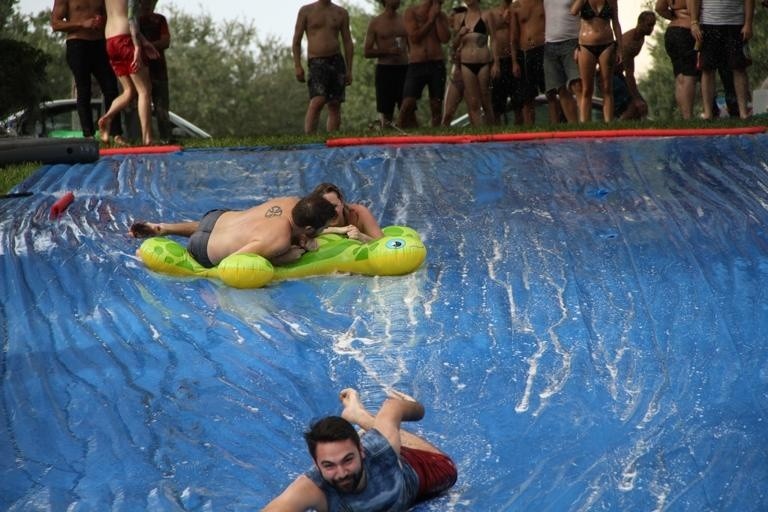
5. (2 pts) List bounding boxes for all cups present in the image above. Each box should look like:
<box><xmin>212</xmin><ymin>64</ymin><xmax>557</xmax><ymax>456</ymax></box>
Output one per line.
<box><xmin>93</xmin><ymin>15</ymin><xmax>106</xmax><ymax>25</ymax></box>
<box><xmin>396</xmin><ymin>36</ymin><xmax>403</xmax><ymax>53</ymax></box>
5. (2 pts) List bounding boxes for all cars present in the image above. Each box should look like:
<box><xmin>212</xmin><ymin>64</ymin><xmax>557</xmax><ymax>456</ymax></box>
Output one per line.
<box><xmin>0</xmin><ymin>96</ymin><xmax>211</xmax><ymax>141</ymax></box>
<box><xmin>434</xmin><ymin>88</ymin><xmax>660</xmax><ymax>128</ymax></box>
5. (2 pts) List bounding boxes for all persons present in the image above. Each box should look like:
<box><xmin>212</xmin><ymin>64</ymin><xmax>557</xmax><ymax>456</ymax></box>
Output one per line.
<box><xmin>136</xmin><ymin>1</ymin><xmax>170</xmax><ymax>141</ymax></box>
<box><xmin>313</xmin><ymin>182</ymin><xmax>385</xmax><ymax>243</ymax></box>
<box><xmin>657</xmin><ymin>1</ymin><xmax>754</xmax><ymax>120</ymax></box>
<box><xmin>98</xmin><ymin>0</ymin><xmax>153</xmax><ymax>144</ymax></box>
<box><xmin>364</xmin><ymin>1</ymin><xmax>451</xmax><ymax>128</ymax></box>
<box><xmin>443</xmin><ymin>1</ymin><xmax>656</xmax><ymax>127</ymax></box>
<box><xmin>260</xmin><ymin>385</ymin><xmax>459</xmax><ymax>512</ymax></box>
<box><xmin>51</xmin><ymin>0</ymin><xmax>129</xmax><ymax>144</ymax></box>
<box><xmin>292</xmin><ymin>1</ymin><xmax>353</xmax><ymax>135</ymax></box>
<box><xmin>129</xmin><ymin>192</ymin><xmax>339</xmax><ymax>267</ymax></box>
